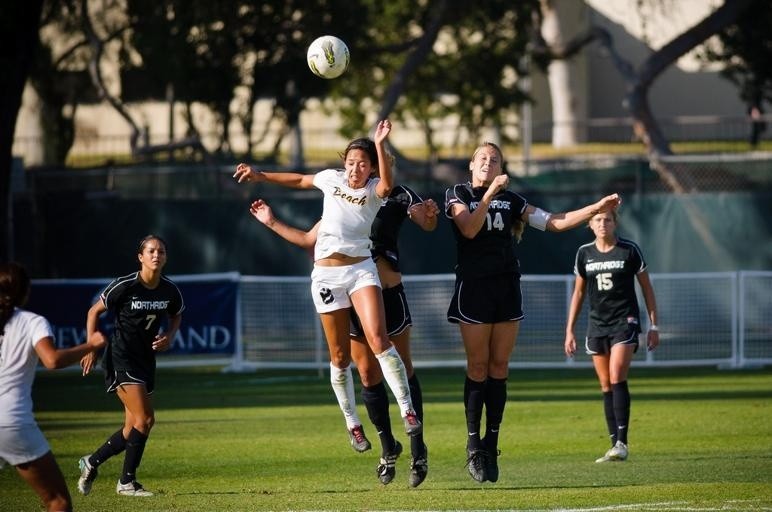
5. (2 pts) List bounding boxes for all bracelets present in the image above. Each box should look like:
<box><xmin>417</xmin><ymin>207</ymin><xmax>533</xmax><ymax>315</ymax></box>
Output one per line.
<box><xmin>649</xmin><ymin>325</ymin><xmax>659</xmax><ymax>332</ymax></box>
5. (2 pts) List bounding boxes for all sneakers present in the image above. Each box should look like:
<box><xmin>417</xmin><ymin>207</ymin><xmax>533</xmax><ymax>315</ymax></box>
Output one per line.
<box><xmin>609</xmin><ymin>440</ymin><xmax>628</xmax><ymax>460</ymax></box>
<box><xmin>402</xmin><ymin>410</ymin><xmax>422</xmax><ymax>437</ymax></box>
<box><xmin>481</xmin><ymin>436</ymin><xmax>501</xmax><ymax>483</ymax></box>
<box><xmin>76</xmin><ymin>454</ymin><xmax>98</xmax><ymax>496</ymax></box>
<box><xmin>376</xmin><ymin>441</ymin><xmax>403</xmax><ymax>485</ymax></box>
<box><xmin>596</xmin><ymin>448</ymin><xmax>617</xmax><ymax>462</ymax></box>
<box><xmin>116</xmin><ymin>478</ymin><xmax>154</xmax><ymax>497</ymax></box>
<box><xmin>408</xmin><ymin>442</ymin><xmax>428</xmax><ymax>487</ymax></box>
<box><xmin>464</xmin><ymin>440</ymin><xmax>488</xmax><ymax>483</ymax></box>
<box><xmin>347</xmin><ymin>424</ymin><xmax>372</xmax><ymax>453</ymax></box>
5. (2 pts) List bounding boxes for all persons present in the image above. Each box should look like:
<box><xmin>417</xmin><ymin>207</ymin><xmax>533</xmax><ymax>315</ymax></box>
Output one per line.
<box><xmin>444</xmin><ymin>140</ymin><xmax>623</xmax><ymax>484</ymax></box>
<box><xmin>232</xmin><ymin>119</ymin><xmax>422</xmax><ymax>452</ymax></box>
<box><xmin>1</xmin><ymin>259</ymin><xmax>110</xmax><ymax>512</ymax></box>
<box><xmin>75</xmin><ymin>233</ymin><xmax>188</xmax><ymax>498</ymax></box>
<box><xmin>249</xmin><ymin>148</ymin><xmax>440</xmax><ymax>487</ymax></box>
<box><xmin>564</xmin><ymin>208</ymin><xmax>660</xmax><ymax>463</ymax></box>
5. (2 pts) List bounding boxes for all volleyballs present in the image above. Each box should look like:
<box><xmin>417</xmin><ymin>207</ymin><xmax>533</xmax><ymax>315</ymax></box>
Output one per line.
<box><xmin>307</xmin><ymin>35</ymin><xmax>351</xmax><ymax>79</ymax></box>
